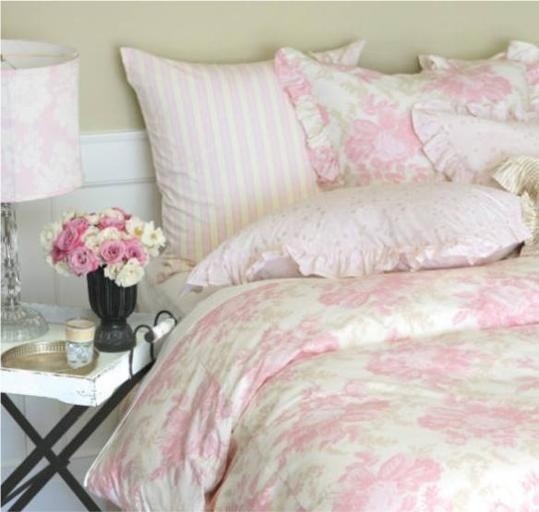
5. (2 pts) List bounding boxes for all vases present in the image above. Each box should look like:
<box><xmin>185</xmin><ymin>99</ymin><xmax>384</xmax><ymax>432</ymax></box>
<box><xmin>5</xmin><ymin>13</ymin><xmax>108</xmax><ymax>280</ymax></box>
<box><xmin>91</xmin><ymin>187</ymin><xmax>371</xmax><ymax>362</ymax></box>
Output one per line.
<box><xmin>86</xmin><ymin>265</ymin><xmax>137</xmax><ymax>352</ymax></box>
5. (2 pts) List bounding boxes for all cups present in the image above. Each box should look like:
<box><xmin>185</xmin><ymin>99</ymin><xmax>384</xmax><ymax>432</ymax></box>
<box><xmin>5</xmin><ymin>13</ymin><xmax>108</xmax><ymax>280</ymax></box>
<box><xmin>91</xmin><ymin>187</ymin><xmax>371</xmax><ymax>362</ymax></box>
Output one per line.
<box><xmin>64</xmin><ymin>316</ymin><xmax>99</xmax><ymax>369</ymax></box>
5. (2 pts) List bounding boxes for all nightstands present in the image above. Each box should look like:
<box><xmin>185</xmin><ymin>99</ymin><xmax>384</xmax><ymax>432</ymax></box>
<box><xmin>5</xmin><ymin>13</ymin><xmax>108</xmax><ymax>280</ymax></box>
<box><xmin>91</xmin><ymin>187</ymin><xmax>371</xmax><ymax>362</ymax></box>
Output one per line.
<box><xmin>0</xmin><ymin>308</ymin><xmax>176</xmax><ymax>511</ymax></box>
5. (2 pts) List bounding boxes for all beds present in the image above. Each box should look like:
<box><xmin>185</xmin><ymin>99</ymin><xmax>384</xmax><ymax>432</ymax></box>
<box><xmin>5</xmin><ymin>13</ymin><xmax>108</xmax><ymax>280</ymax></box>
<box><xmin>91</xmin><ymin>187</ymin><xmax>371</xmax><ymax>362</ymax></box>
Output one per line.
<box><xmin>13</xmin><ymin>130</ymin><xmax>538</xmax><ymax>512</ymax></box>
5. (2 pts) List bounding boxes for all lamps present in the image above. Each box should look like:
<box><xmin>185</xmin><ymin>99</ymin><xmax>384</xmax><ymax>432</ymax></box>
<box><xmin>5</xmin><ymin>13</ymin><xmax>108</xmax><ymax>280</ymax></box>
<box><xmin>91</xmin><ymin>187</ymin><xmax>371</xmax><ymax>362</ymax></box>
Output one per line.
<box><xmin>0</xmin><ymin>39</ymin><xmax>83</xmax><ymax>343</ymax></box>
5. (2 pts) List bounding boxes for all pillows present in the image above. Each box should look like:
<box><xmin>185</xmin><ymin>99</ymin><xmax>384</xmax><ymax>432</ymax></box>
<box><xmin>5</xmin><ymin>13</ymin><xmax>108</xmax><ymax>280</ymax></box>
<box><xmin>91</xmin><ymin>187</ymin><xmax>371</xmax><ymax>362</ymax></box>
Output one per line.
<box><xmin>188</xmin><ymin>182</ymin><xmax>537</xmax><ymax>300</ymax></box>
<box><xmin>119</xmin><ymin>35</ymin><xmax>366</xmax><ymax>284</ymax></box>
<box><xmin>411</xmin><ymin>100</ymin><xmax>539</xmax><ymax>200</ymax></box>
<box><xmin>272</xmin><ymin>40</ymin><xmax>539</xmax><ymax>190</ymax></box>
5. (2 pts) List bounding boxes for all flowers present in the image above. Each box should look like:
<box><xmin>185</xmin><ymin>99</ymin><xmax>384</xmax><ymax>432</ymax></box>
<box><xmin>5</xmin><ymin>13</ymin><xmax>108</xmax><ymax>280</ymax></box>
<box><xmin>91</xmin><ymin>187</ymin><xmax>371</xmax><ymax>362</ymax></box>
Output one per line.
<box><xmin>39</xmin><ymin>204</ymin><xmax>166</xmax><ymax>289</ymax></box>
